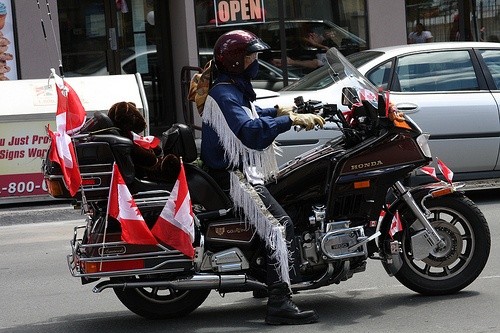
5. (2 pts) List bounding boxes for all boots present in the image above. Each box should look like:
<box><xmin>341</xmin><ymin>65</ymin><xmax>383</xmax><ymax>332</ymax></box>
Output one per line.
<box><xmin>265</xmin><ymin>282</ymin><xmax>320</xmax><ymax>325</ymax></box>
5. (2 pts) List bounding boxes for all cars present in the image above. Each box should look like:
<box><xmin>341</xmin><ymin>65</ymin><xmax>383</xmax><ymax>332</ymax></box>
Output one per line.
<box><xmin>63</xmin><ymin>46</ymin><xmax>301</xmax><ymax>92</ymax></box>
<box><xmin>197</xmin><ymin>19</ymin><xmax>367</xmax><ymax>74</ymax></box>
<box><xmin>251</xmin><ymin>41</ymin><xmax>500</xmax><ymax>201</ymax></box>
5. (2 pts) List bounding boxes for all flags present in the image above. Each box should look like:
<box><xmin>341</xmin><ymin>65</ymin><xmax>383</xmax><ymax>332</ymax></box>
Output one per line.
<box><xmin>151</xmin><ymin>156</ymin><xmax>196</xmax><ymax>259</ymax></box>
<box><xmin>106</xmin><ymin>161</ymin><xmax>160</xmax><ymax>245</ymax></box>
<box><xmin>47</xmin><ymin>67</ymin><xmax>88</xmax><ymax>136</ymax></box>
<box><xmin>44</xmin><ymin>125</ymin><xmax>83</xmax><ymax>196</ymax></box>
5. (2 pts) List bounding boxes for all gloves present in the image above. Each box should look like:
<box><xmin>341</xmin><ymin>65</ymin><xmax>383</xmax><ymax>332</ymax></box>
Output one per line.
<box><xmin>273</xmin><ymin>102</ymin><xmax>299</xmax><ymax>117</ymax></box>
<box><xmin>288</xmin><ymin>111</ymin><xmax>326</xmax><ymax>132</ymax></box>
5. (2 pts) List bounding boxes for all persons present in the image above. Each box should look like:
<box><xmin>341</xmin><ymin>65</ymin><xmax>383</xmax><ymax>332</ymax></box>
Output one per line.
<box><xmin>479</xmin><ymin>26</ymin><xmax>486</xmax><ymax>42</ymax></box>
<box><xmin>408</xmin><ymin>22</ymin><xmax>433</xmax><ymax>44</ymax></box>
<box><xmin>197</xmin><ymin>28</ymin><xmax>326</xmax><ymax>326</ymax></box>
<box><xmin>271</xmin><ymin>26</ymin><xmax>339</xmax><ymax>70</ymax></box>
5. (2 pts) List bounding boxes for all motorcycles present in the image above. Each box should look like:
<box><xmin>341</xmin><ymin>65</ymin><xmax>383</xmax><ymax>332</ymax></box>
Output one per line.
<box><xmin>41</xmin><ymin>46</ymin><xmax>492</xmax><ymax>320</ymax></box>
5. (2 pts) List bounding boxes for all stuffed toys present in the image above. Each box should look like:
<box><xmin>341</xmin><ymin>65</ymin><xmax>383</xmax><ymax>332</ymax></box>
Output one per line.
<box><xmin>107</xmin><ymin>102</ymin><xmax>147</xmax><ymax>136</ymax></box>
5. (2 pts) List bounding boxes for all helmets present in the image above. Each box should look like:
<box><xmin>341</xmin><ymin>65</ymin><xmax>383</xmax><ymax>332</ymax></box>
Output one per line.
<box><xmin>214</xmin><ymin>30</ymin><xmax>271</xmax><ymax>75</ymax></box>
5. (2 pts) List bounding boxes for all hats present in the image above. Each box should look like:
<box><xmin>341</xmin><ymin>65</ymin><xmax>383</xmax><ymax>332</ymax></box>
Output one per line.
<box><xmin>187</xmin><ymin>59</ymin><xmax>212</xmax><ymax>118</ymax></box>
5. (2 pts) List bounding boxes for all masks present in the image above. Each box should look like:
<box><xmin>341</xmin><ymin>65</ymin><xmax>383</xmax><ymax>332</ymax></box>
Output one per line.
<box><xmin>243</xmin><ymin>59</ymin><xmax>259</xmax><ymax>80</ymax></box>
